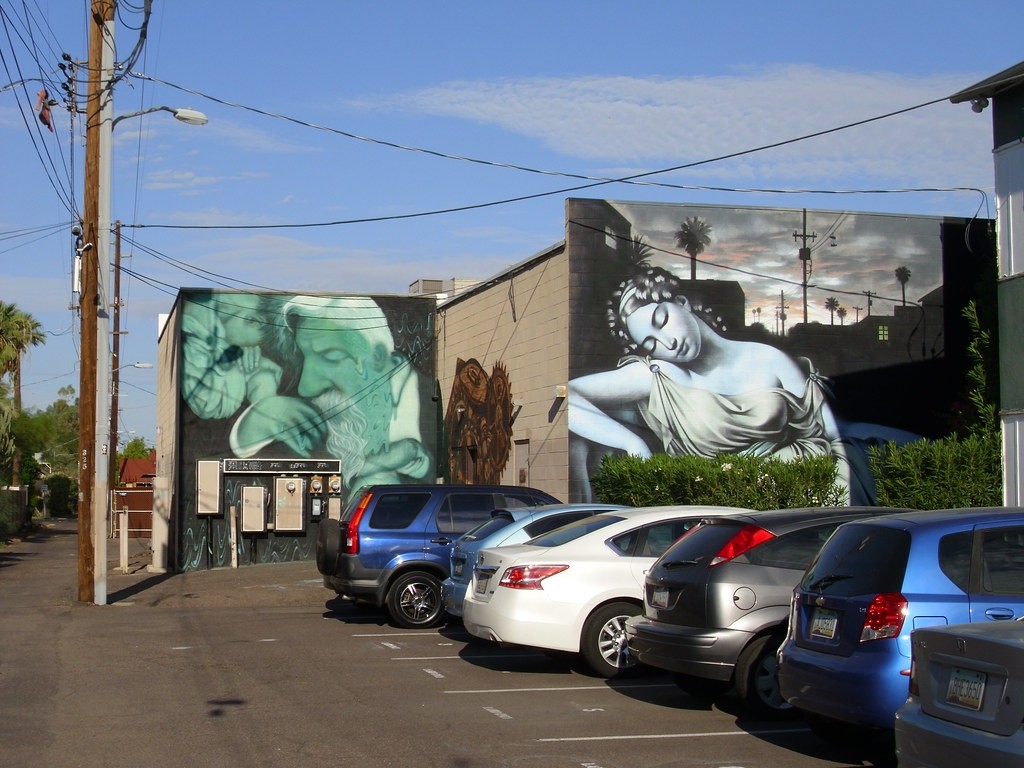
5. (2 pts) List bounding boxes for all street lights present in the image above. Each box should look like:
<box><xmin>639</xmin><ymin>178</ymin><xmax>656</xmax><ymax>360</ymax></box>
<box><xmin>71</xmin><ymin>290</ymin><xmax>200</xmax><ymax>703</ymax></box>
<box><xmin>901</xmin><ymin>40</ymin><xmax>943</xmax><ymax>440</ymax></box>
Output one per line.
<box><xmin>91</xmin><ymin>107</ymin><xmax>209</xmax><ymax>609</ymax></box>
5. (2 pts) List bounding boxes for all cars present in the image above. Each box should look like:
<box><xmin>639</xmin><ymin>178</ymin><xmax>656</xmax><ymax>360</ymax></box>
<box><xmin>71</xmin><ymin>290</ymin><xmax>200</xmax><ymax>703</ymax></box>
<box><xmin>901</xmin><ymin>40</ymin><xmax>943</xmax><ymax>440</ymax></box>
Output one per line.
<box><xmin>443</xmin><ymin>502</ymin><xmax>757</xmax><ymax>680</ymax></box>
<box><xmin>894</xmin><ymin>618</ymin><xmax>1024</xmax><ymax>768</ymax></box>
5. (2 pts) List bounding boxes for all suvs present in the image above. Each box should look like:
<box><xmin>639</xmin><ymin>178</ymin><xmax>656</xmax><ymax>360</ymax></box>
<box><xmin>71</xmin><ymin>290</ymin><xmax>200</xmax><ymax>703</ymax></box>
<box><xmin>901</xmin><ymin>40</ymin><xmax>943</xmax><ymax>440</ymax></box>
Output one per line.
<box><xmin>624</xmin><ymin>508</ymin><xmax>921</xmax><ymax>724</ymax></box>
<box><xmin>318</xmin><ymin>483</ymin><xmax>565</xmax><ymax>626</ymax></box>
<box><xmin>778</xmin><ymin>504</ymin><xmax>1024</xmax><ymax>739</ymax></box>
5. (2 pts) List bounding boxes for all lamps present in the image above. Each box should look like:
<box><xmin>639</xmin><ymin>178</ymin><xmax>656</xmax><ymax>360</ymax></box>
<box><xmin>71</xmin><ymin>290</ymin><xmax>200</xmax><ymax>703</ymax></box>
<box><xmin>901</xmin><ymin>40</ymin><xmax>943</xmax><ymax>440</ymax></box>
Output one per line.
<box><xmin>970</xmin><ymin>94</ymin><xmax>989</xmax><ymax>114</ymax></box>
